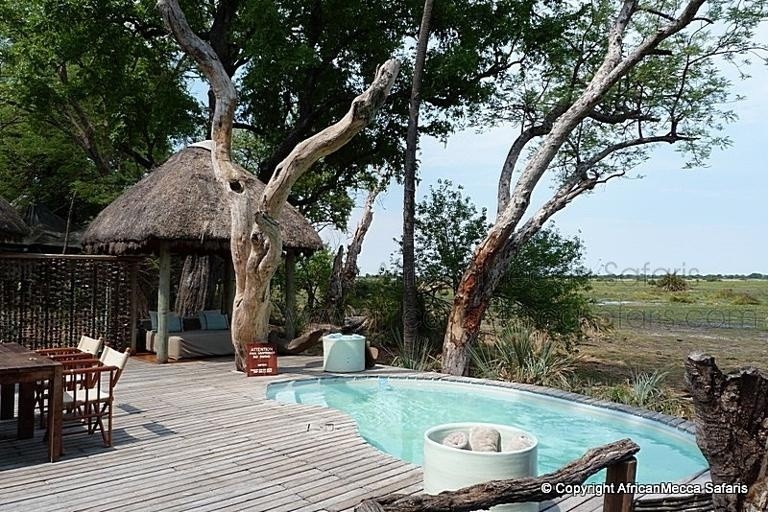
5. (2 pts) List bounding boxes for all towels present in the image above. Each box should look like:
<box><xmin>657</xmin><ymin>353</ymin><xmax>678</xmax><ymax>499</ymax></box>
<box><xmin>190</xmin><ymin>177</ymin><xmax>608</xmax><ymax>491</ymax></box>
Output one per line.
<box><xmin>438</xmin><ymin>426</ymin><xmax>532</xmax><ymax>454</ymax></box>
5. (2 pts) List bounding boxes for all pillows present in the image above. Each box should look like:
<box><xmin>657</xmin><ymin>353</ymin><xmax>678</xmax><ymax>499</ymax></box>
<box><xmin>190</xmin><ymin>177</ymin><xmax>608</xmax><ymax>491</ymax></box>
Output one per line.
<box><xmin>147</xmin><ymin>309</ymin><xmax>230</xmax><ymax>334</ymax></box>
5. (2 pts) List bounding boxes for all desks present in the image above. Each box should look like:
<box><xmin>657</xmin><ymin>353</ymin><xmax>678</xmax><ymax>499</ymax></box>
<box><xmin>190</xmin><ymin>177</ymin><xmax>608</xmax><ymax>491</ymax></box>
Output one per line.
<box><xmin>1</xmin><ymin>341</ymin><xmax>63</xmax><ymax>463</ymax></box>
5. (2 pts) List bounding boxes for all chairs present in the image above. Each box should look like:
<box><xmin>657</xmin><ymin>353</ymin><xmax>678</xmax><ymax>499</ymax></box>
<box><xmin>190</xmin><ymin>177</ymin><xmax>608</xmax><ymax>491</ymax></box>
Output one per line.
<box><xmin>34</xmin><ymin>334</ymin><xmax>133</xmax><ymax>447</ymax></box>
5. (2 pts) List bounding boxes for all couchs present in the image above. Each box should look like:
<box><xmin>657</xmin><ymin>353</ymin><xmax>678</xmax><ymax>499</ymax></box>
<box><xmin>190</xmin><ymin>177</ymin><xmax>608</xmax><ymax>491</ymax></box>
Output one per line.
<box><xmin>139</xmin><ymin>319</ymin><xmax>235</xmax><ymax>361</ymax></box>
